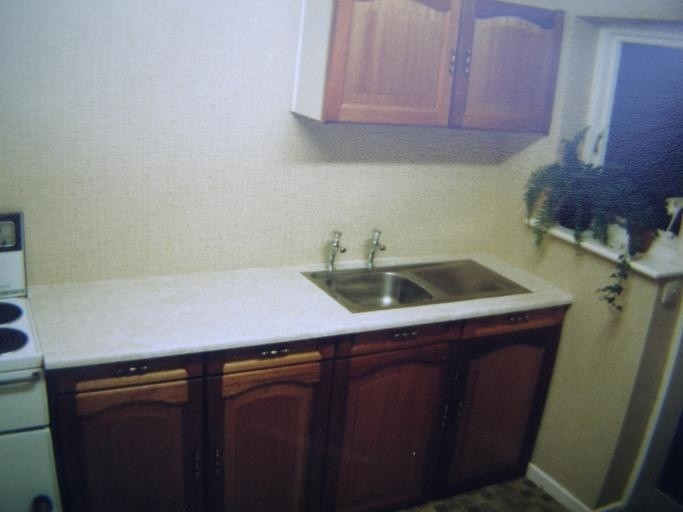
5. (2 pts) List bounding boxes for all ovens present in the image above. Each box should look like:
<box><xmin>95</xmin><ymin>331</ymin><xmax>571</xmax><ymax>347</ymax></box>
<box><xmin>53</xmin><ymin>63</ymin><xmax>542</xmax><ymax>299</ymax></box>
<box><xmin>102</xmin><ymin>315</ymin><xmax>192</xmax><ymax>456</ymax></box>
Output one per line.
<box><xmin>0</xmin><ymin>372</ymin><xmax>63</xmax><ymax>510</ymax></box>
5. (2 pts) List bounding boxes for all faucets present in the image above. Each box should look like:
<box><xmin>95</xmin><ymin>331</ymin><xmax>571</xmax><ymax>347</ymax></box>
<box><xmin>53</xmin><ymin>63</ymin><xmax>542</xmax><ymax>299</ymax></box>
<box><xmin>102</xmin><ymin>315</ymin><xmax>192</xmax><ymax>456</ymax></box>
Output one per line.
<box><xmin>327</xmin><ymin>231</ymin><xmax>347</xmax><ymax>271</ymax></box>
<box><xmin>367</xmin><ymin>229</ymin><xmax>386</xmax><ymax>270</ymax></box>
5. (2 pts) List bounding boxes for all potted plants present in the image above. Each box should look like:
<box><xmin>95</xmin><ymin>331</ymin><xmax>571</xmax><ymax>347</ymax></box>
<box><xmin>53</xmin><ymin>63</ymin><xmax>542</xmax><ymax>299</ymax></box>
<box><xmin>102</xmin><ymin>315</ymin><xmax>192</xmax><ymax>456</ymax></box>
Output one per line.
<box><xmin>591</xmin><ymin>206</ymin><xmax>660</xmax><ymax>319</ymax></box>
<box><xmin>515</xmin><ymin>118</ymin><xmax>653</xmax><ymax>259</ymax></box>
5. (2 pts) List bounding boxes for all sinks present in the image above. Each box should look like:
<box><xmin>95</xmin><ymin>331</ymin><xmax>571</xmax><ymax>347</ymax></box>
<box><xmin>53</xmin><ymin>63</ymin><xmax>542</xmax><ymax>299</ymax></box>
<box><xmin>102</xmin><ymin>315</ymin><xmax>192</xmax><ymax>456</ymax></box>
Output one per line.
<box><xmin>409</xmin><ymin>261</ymin><xmax>533</xmax><ymax>305</ymax></box>
<box><xmin>298</xmin><ymin>265</ymin><xmax>434</xmax><ymax>317</ymax></box>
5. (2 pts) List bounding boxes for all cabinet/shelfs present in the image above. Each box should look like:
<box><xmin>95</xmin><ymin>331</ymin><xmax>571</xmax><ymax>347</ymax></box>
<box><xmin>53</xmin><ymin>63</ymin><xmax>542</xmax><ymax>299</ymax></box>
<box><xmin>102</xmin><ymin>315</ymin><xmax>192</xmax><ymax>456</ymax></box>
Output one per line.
<box><xmin>39</xmin><ymin>333</ymin><xmax>335</xmax><ymax>511</ymax></box>
<box><xmin>326</xmin><ymin>302</ymin><xmax>571</xmax><ymax>511</ymax></box>
<box><xmin>291</xmin><ymin>0</ymin><xmax>568</xmax><ymax>139</ymax></box>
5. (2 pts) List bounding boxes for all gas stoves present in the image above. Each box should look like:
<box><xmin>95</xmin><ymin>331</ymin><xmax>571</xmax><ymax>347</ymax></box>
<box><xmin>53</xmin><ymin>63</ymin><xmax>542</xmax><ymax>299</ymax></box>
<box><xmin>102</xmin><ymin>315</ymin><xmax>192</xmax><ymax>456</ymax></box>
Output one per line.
<box><xmin>0</xmin><ymin>292</ymin><xmax>43</xmax><ymax>371</ymax></box>
